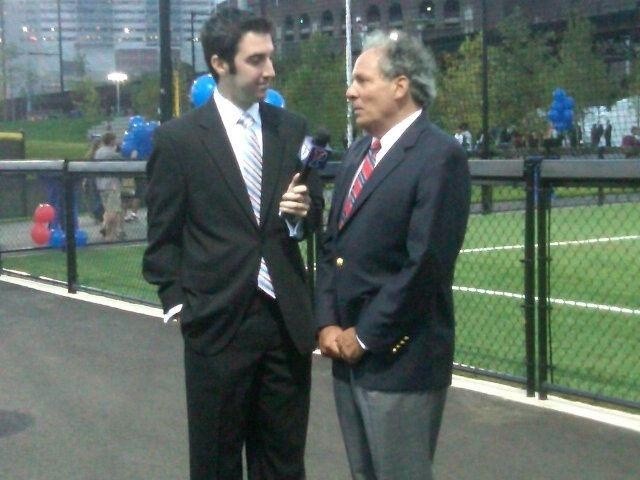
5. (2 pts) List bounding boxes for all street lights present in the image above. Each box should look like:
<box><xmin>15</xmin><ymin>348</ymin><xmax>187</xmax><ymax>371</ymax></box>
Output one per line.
<box><xmin>106</xmin><ymin>71</ymin><xmax>129</xmax><ymax>115</ymax></box>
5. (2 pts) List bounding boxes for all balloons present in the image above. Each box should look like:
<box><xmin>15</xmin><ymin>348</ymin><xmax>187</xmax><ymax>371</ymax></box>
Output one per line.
<box><xmin>190</xmin><ymin>73</ymin><xmax>216</xmax><ymax>108</ymax></box>
<box><xmin>69</xmin><ymin>228</ymin><xmax>90</xmax><ymax>246</ymax></box>
<box><xmin>47</xmin><ymin>225</ymin><xmax>69</xmax><ymax>248</ymax></box>
<box><xmin>33</xmin><ymin>203</ymin><xmax>56</xmax><ymax>223</ymax></box>
<box><xmin>120</xmin><ymin>116</ymin><xmax>164</xmax><ymax>163</ymax></box>
<box><xmin>29</xmin><ymin>223</ymin><xmax>53</xmax><ymax>244</ymax></box>
<box><xmin>260</xmin><ymin>89</ymin><xmax>286</xmax><ymax>110</ymax></box>
<box><xmin>545</xmin><ymin>87</ymin><xmax>577</xmax><ymax>135</ymax></box>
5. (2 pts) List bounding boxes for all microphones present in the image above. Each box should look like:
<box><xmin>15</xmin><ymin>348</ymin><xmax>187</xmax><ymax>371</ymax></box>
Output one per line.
<box><xmin>278</xmin><ymin>128</ymin><xmax>334</xmax><ymax>222</ymax></box>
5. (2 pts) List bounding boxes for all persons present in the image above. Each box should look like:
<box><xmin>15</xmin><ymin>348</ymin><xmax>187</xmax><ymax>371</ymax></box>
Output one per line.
<box><xmin>452</xmin><ymin>121</ymin><xmax>585</xmax><ymax>156</ymax></box>
<box><xmin>590</xmin><ymin>124</ymin><xmax>598</xmax><ymax>147</ymax></box>
<box><xmin>621</xmin><ymin>126</ymin><xmax>640</xmax><ymax>158</ymax></box>
<box><xmin>138</xmin><ymin>5</ymin><xmax>328</xmax><ymax>480</ymax></box>
<box><xmin>289</xmin><ymin>27</ymin><xmax>474</xmax><ymax>480</ymax></box>
<box><xmin>120</xmin><ymin>175</ymin><xmax>143</xmax><ymax>225</ymax></box>
<box><xmin>596</xmin><ymin>121</ymin><xmax>604</xmax><ymax>146</ymax></box>
<box><xmin>604</xmin><ymin>119</ymin><xmax>612</xmax><ymax>147</ymax></box>
<box><xmin>90</xmin><ymin>131</ymin><xmax>130</xmax><ymax>243</ymax></box>
<box><xmin>80</xmin><ymin>142</ymin><xmax>108</xmax><ymax>224</ymax></box>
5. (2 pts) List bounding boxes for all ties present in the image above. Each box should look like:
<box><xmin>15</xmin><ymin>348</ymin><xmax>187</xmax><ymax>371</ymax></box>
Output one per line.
<box><xmin>339</xmin><ymin>141</ymin><xmax>381</xmax><ymax>229</ymax></box>
<box><xmin>236</xmin><ymin>113</ymin><xmax>276</xmax><ymax>300</ymax></box>
